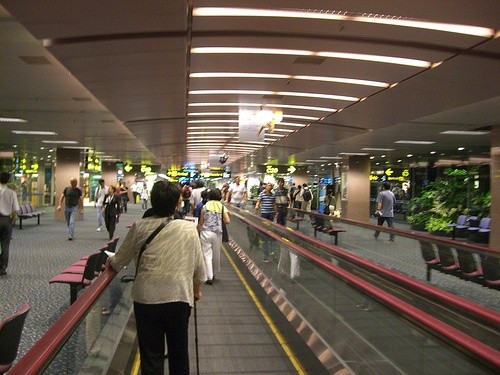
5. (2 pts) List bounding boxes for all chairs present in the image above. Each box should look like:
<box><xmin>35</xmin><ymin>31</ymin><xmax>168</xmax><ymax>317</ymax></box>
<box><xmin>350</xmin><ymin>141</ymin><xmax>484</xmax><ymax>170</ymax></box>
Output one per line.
<box><xmin>0</xmin><ymin>304</ymin><xmax>32</xmax><ymax>375</ymax></box>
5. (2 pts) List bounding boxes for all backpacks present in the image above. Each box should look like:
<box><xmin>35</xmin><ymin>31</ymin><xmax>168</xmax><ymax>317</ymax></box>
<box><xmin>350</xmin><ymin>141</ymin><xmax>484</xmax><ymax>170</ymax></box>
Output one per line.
<box><xmin>303</xmin><ymin>188</ymin><xmax>311</xmax><ymax>201</ymax></box>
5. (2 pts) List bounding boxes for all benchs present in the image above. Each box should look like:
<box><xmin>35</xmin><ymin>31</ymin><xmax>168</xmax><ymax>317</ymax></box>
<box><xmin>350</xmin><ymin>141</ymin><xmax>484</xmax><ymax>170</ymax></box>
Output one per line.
<box><xmin>285</xmin><ymin>213</ymin><xmax>304</xmax><ymax>230</ymax></box>
<box><xmin>308</xmin><ymin>214</ymin><xmax>344</xmax><ymax>246</ymax></box>
<box><xmin>449</xmin><ymin>215</ymin><xmax>492</xmax><ymax>241</ymax></box>
<box><xmin>419</xmin><ymin>241</ymin><xmax>500</xmax><ymax>291</ymax></box>
<box><xmin>49</xmin><ymin>236</ymin><xmax>119</xmax><ymax>305</ymax></box>
<box><xmin>18</xmin><ymin>203</ymin><xmax>47</xmax><ymax>230</ymax></box>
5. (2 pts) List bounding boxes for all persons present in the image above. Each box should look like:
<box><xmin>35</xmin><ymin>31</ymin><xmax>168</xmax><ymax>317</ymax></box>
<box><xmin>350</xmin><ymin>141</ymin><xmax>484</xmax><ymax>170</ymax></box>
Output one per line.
<box><xmin>140</xmin><ymin>179</ymin><xmax>211</xmax><ymax>220</ymax></box>
<box><xmin>197</xmin><ymin>188</ymin><xmax>230</xmax><ymax>284</ymax></box>
<box><xmin>95</xmin><ymin>179</ymin><xmax>109</xmax><ymax>231</ymax></box>
<box><xmin>103</xmin><ymin>182</ymin><xmax>130</xmax><ymax>240</ymax></box>
<box><xmin>222</xmin><ymin>177</ymin><xmax>244</xmax><ymax>208</ymax></box>
<box><xmin>290</xmin><ymin>182</ymin><xmax>313</xmax><ymax>223</ymax></box>
<box><xmin>255</xmin><ymin>182</ymin><xmax>276</xmax><ymax>222</ymax></box>
<box><xmin>0</xmin><ymin>172</ymin><xmax>20</xmax><ymax>275</ymax></box>
<box><xmin>374</xmin><ymin>183</ymin><xmax>396</xmax><ymax>242</ymax></box>
<box><xmin>130</xmin><ymin>182</ymin><xmax>139</xmax><ymax>204</ymax></box>
<box><xmin>324</xmin><ymin>191</ymin><xmax>336</xmax><ymax>215</ymax></box>
<box><xmin>106</xmin><ymin>180</ymin><xmax>208</xmax><ymax>375</ymax></box>
<box><xmin>273</xmin><ymin>179</ymin><xmax>288</xmax><ymax>225</ymax></box>
<box><xmin>57</xmin><ymin>178</ymin><xmax>84</xmax><ymax>240</ymax></box>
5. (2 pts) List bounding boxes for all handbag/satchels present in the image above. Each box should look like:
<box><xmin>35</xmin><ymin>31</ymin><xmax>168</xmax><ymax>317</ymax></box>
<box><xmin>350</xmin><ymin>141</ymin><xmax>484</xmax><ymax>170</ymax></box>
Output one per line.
<box><xmin>222</xmin><ymin>223</ymin><xmax>229</xmax><ymax>242</ymax></box>
<box><xmin>325</xmin><ymin>207</ymin><xmax>330</xmax><ymax>215</ymax></box>
<box><xmin>293</xmin><ymin>196</ymin><xmax>296</xmax><ymax>199</ymax></box>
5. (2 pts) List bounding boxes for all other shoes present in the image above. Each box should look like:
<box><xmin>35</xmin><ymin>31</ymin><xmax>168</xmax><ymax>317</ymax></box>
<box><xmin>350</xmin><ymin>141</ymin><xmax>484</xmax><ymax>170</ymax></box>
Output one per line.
<box><xmin>270</xmin><ymin>252</ymin><xmax>277</xmax><ymax>261</ymax></box>
<box><xmin>263</xmin><ymin>258</ymin><xmax>270</xmax><ymax>263</ymax></box>
<box><xmin>206</xmin><ymin>276</ymin><xmax>214</xmax><ymax>285</ymax></box>
<box><xmin>97</xmin><ymin>227</ymin><xmax>103</xmax><ymax>232</ymax></box>
<box><xmin>68</xmin><ymin>236</ymin><xmax>73</xmax><ymax>241</ymax></box>
